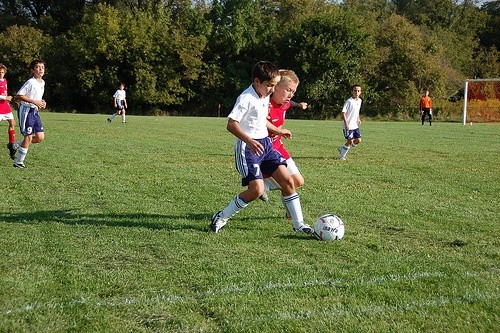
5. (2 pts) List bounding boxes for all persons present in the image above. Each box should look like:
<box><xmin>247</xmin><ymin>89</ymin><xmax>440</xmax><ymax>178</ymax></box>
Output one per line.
<box><xmin>210</xmin><ymin>61</ymin><xmax>316</xmax><ymax>237</ymax></box>
<box><xmin>105</xmin><ymin>82</ymin><xmax>128</xmax><ymax>124</ymax></box>
<box><xmin>420</xmin><ymin>90</ymin><xmax>433</xmax><ymax>126</ymax></box>
<box><xmin>7</xmin><ymin>59</ymin><xmax>47</xmax><ymax>170</ymax></box>
<box><xmin>247</xmin><ymin>69</ymin><xmax>304</xmax><ymax>201</ymax></box>
<box><xmin>0</xmin><ymin>63</ymin><xmax>15</xmax><ymax>144</ymax></box>
<box><xmin>337</xmin><ymin>84</ymin><xmax>362</xmax><ymax>160</ymax></box>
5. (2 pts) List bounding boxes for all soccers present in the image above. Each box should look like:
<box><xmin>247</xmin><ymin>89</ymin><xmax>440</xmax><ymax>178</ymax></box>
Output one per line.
<box><xmin>314</xmin><ymin>213</ymin><xmax>344</xmax><ymax>240</ymax></box>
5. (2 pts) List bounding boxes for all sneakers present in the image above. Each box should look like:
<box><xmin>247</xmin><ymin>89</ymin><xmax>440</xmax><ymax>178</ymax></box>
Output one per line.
<box><xmin>13</xmin><ymin>163</ymin><xmax>26</xmax><ymax>168</ymax></box>
<box><xmin>6</xmin><ymin>142</ymin><xmax>17</xmax><ymax>160</ymax></box>
<box><xmin>210</xmin><ymin>211</ymin><xmax>226</xmax><ymax>233</ymax></box>
<box><xmin>293</xmin><ymin>223</ymin><xmax>315</xmax><ymax>235</ymax></box>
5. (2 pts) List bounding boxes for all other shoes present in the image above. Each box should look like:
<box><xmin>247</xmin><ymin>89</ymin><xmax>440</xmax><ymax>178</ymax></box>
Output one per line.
<box><xmin>107</xmin><ymin>118</ymin><xmax>111</xmax><ymax>122</ymax></box>
<box><xmin>282</xmin><ymin>212</ymin><xmax>292</xmax><ymax>221</ymax></box>
<box><xmin>338</xmin><ymin>156</ymin><xmax>347</xmax><ymax>160</ymax></box>
<box><xmin>338</xmin><ymin>146</ymin><xmax>343</xmax><ymax>154</ymax></box>
<box><xmin>259</xmin><ymin>191</ymin><xmax>268</xmax><ymax>202</ymax></box>
